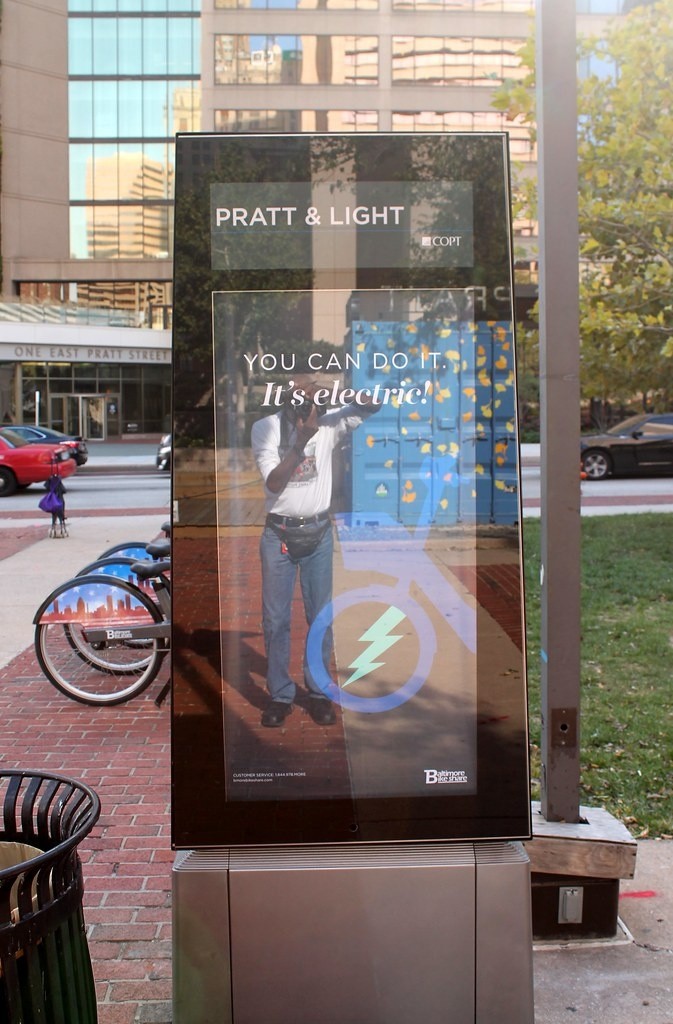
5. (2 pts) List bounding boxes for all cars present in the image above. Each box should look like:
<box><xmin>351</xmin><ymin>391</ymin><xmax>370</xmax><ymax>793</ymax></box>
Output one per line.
<box><xmin>3</xmin><ymin>426</ymin><xmax>89</xmax><ymax>467</ymax></box>
<box><xmin>580</xmin><ymin>413</ymin><xmax>673</xmax><ymax>482</ymax></box>
<box><xmin>1</xmin><ymin>427</ymin><xmax>78</xmax><ymax>501</ymax></box>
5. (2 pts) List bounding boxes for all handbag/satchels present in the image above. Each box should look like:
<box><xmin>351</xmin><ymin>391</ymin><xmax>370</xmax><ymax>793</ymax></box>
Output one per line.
<box><xmin>37</xmin><ymin>475</ymin><xmax>64</xmax><ymax>513</ymax></box>
<box><xmin>270</xmin><ymin>522</ymin><xmax>329</xmax><ymax>559</ymax></box>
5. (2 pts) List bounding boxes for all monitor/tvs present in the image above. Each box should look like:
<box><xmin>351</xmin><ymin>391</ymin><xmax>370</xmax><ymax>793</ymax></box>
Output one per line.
<box><xmin>168</xmin><ymin>131</ymin><xmax>536</xmax><ymax>846</ymax></box>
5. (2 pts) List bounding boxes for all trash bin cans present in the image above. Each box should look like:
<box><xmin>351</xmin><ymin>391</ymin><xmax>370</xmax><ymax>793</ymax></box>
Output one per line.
<box><xmin>0</xmin><ymin>767</ymin><xmax>108</xmax><ymax>1024</ymax></box>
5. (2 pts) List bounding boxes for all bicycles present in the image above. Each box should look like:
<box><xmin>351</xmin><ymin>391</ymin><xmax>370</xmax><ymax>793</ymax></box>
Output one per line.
<box><xmin>32</xmin><ymin>521</ymin><xmax>170</xmax><ymax>711</ymax></box>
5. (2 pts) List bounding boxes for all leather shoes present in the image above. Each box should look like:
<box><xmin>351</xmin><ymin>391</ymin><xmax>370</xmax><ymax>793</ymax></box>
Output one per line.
<box><xmin>310</xmin><ymin>696</ymin><xmax>339</xmax><ymax>727</ymax></box>
<box><xmin>262</xmin><ymin>701</ymin><xmax>293</xmax><ymax>727</ymax></box>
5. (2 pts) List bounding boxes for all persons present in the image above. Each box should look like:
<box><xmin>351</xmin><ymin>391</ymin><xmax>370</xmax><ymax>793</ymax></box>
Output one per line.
<box><xmin>253</xmin><ymin>370</ymin><xmax>384</xmax><ymax>734</ymax></box>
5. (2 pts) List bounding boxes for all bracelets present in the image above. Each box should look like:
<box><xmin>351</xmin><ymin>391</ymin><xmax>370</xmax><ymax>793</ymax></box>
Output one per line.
<box><xmin>293</xmin><ymin>445</ymin><xmax>304</xmax><ymax>455</ymax></box>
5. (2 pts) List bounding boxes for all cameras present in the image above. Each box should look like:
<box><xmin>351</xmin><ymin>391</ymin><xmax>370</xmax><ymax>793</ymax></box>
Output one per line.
<box><xmin>291</xmin><ymin>397</ymin><xmax>326</xmax><ymax>423</ymax></box>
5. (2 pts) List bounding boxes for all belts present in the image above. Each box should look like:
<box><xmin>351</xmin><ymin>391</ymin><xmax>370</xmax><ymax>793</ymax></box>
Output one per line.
<box><xmin>266</xmin><ymin>513</ymin><xmax>331</xmax><ymax>527</ymax></box>
